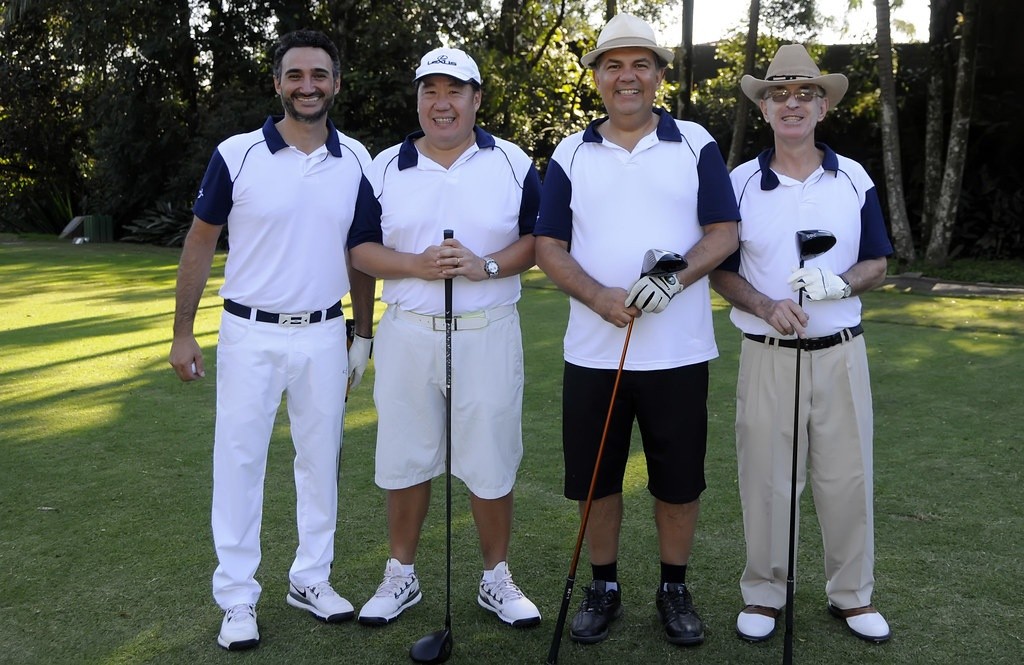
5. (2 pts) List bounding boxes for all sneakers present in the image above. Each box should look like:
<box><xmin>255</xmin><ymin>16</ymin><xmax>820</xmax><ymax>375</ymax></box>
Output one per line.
<box><xmin>218</xmin><ymin>604</ymin><xmax>260</xmax><ymax>651</ymax></box>
<box><xmin>656</xmin><ymin>583</ymin><xmax>704</xmax><ymax>645</ymax></box>
<box><xmin>477</xmin><ymin>561</ymin><xmax>542</xmax><ymax>628</ymax></box>
<box><xmin>287</xmin><ymin>582</ymin><xmax>354</xmax><ymax>622</ymax></box>
<box><xmin>735</xmin><ymin>604</ymin><xmax>779</xmax><ymax>643</ymax></box>
<box><xmin>570</xmin><ymin>580</ymin><xmax>621</xmax><ymax>644</ymax></box>
<box><xmin>828</xmin><ymin>601</ymin><xmax>891</xmax><ymax>643</ymax></box>
<box><xmin>358</xmin><ymin>558</ymin><xmax>422</xmax><ymax>625</ymax></box>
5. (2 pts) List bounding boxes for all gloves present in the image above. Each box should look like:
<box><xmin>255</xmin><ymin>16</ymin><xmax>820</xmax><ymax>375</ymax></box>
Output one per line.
<box><xmin>787</xmin><ymin>267</ymin><xmax>845</xmax><ymax>301</ymax></box>
<box><xmin>625</xmin><ymin>273</ymin><xmax>684</xmax><ymax>313</ymax></box>
<box><xmin>348</xmin><ymin>334</ymin><xmax>374</xmax><ymax>394</ymax></box>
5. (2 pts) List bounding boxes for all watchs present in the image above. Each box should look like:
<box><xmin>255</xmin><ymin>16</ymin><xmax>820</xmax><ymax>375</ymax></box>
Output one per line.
<box><xmin>483</xmin><ymin>256</ymin><xmax>499</xmax><ymax>280</ymax></box>
<box><xmin>838</xmin><ymin>274</ymin><xmax>852</xmax><ymax>300</ymax></box>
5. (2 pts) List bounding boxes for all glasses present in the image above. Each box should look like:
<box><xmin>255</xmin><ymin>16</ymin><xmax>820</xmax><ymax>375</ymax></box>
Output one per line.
<box><xmin>765</xmin><ymin>85</ymin><xmax>823</xmax><ymax>103</ymax></box>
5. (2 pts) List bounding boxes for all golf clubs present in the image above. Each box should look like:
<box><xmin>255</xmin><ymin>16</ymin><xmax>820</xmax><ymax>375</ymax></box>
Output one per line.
<box><xmin>408</xmin><ymin>227</ymin><xmax>458</xmax><ymax>664</ymax></box>
<box><xmin>781</xmin><ymin>228</ymin><xmax>839</xmax><ymax>665</ymax></box>
<box><xmin>545</xmin><ymin>245</ymin><xmax>689</xmax><ymax>665</ymax></box>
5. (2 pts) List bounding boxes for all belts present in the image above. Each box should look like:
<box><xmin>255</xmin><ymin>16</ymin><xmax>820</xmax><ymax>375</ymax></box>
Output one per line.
<box><xmin>224</xmin><ymin>298</ymin><xmax>344</xmax><ymax>324</ymax></box>
<box><xmin>387</xmin><ymin>303</ymin><xmax>515</xmax><ymax>331</ymax></box>
<box><xmin>744</xmin><ymin>324</ymin><xmax>864</xmax><ymax>351</ymax></box>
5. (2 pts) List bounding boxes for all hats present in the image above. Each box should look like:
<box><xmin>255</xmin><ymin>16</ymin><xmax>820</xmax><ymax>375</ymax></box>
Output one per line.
<box><xmin>412</xmin><ymin>47</ymin><xmax>482</xmax><ymax>86</ymax></box>
<box><xmin>580</xmin><ymin>14</ymin><xmax>674</xmax><ymax>69</ymax></box>
<box><xmin>740</xmin><ymin>45</ymin><xmax>849</xmax><ymax>110</ymax></box>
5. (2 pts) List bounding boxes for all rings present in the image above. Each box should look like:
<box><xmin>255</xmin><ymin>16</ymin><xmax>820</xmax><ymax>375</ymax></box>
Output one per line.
<box><xmin>457</xmin><ymin>257</ymin><xmax>459</xmax><ymax>266</ymax></box>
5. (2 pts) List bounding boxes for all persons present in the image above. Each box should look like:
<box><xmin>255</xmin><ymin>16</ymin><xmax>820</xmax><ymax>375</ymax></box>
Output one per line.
<box><xmin>167</xmin><ymin>29</ymin><xmax>376</xmax><ymax>652</ymax></box>
<box><xmin>534</xmin><ymin>14</ymin><xmax>741</xmax><ymax>646</ymax></box>
<box><xmin>707</xmin><ymin>45</ymin><xmax>894</xmax><ymax>641</ymax></box>
<box><xmin>347</xmin><ymin>48</ymin><xmax>542</xmax><ymax>630</ymax></box>
